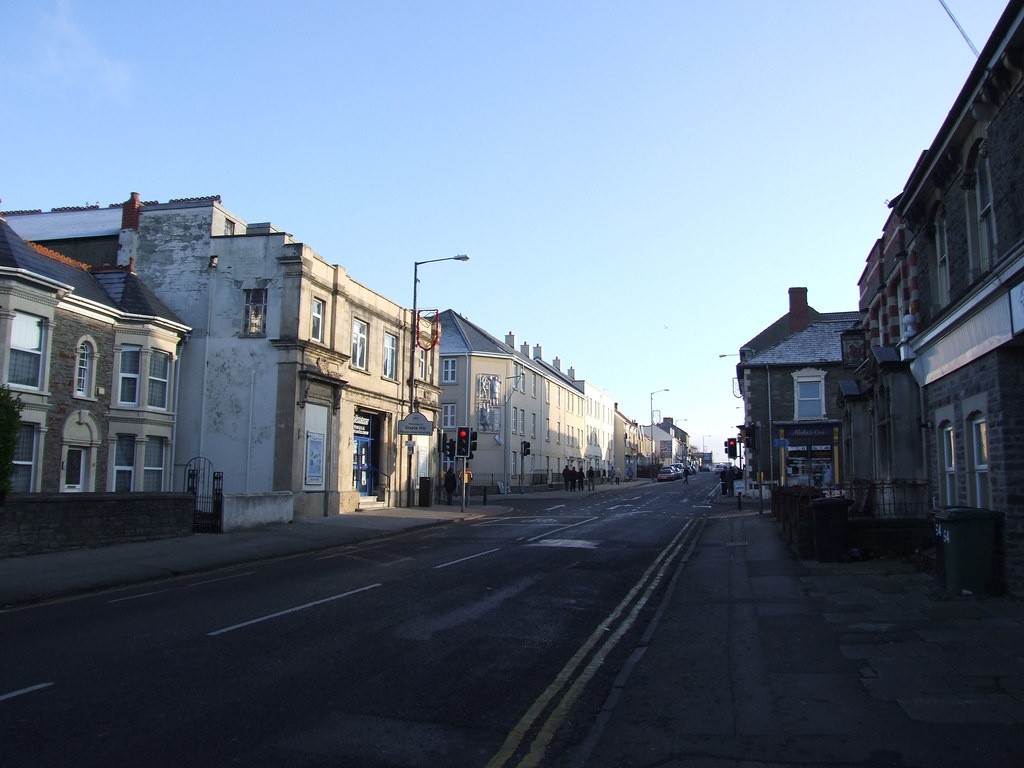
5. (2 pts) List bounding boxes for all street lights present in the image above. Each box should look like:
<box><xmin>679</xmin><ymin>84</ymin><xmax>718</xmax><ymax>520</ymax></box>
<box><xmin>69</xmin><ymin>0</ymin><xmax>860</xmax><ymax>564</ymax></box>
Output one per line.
<box><xmin>406</xmin><ymin>254</ymin><xmax>470</xmax><ymax>505</ymax></box>
<box><xmin>651</xmin><ymin>389</ymin><xmax>670</xmax><ymax>480</ymax></box>
<box><xmin>719</xmin><ymin>353</ymin><xmax>774</xmax><ymax>490</ymax></box>
<box><xmin>702</xmin><ymin>435</ymin><xmax>711</xmax><ymax>452</ymax></box>
<box><xmin>675</xmin><ymin>418</ymin><xmax>688</xmax><ymax>426</ymax></box>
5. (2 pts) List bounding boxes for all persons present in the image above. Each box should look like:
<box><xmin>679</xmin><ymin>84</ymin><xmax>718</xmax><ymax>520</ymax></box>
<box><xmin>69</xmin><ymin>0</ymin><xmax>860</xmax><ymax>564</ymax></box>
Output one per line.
<box><xmin>683</xmin><ymin>465</ymin><xmax>689</xmax><ymax>485</ymax></box>
<box><xmin>812</xmin><ymin>468</ymin><xmax>823</xmax><ymax>487</ymax></box>
<box><xmin>562</xmin><ymin>465</ymin><xmax>641</xmax><ymax>492</ymax></box>
<box><xmin>720</xmin><ymin>466</ymin><xmax>742</xmax><ymax>497</ymax></box>
<box><xmin>443</xmin><ymin>461</ymin><xmax>473</xmax><ymax>507</ymax></box>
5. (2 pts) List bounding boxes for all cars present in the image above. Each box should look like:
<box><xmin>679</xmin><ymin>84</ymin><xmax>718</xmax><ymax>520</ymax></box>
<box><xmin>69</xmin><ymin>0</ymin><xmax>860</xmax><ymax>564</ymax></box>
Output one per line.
<box><xmin>713</xmin><ymin>464</ymin><xmax>727</xmax><ymax>475</ymax></box>
<box><xmin>666</xmin><ymin>461</ymin><xmax>712</xmax><ymax>479</ymax></box>
<box><xmin>656</xmin><ymin>468</ymin><xmax>675</xmax><ymax>482</ymax></box>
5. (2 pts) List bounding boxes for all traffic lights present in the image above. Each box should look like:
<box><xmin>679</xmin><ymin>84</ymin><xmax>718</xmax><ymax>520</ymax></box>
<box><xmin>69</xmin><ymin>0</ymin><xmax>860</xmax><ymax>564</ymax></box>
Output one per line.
<box><xmin>523</xmin><ymin>441</ymin><xmax>531</xmax><ymax>456</ymax></box>
<box><xmin>446</xmin><ymin>437</ymin><xmax>455</xmax><ymax>459</ymax></box>
<box><xmin>727</xmin><ymin>438</ymin><xmax>738</xmax><ymax>459</ymax></box>
<box><xmin>455</xmin><ymin>426</ymin><xmax>471</xmax><ymax>458</ymax></box>
<box><xmin>470</xmin><ymin>427</ymin><xmax>478</xmax><ymax>458</ymax></box>
<box><xmin>724</xmin><ymin>440</ymin><xmax>728</xmax><ymax>454</ymax></box>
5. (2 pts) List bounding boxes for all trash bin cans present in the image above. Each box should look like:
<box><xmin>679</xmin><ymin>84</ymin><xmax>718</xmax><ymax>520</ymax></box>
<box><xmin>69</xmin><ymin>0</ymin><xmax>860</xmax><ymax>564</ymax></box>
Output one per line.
<box><xmin>933</xmin><ymin>508</ymin><xmax>1005</xmax><ymax>601</ymax></box>
<box><xmin>929</xmin><ymin>505</ymin><xmax>973</xmax><ymax>594</ymax></box>
<box><xmin>419</xmin><ymin>477</ymin><xmax>434</xmax><ymax>508</ymax></box>
<box><xmin>807</xmin><ymin>495</ymin><xmax>855</xmax><ymax>564</ymax></box>
